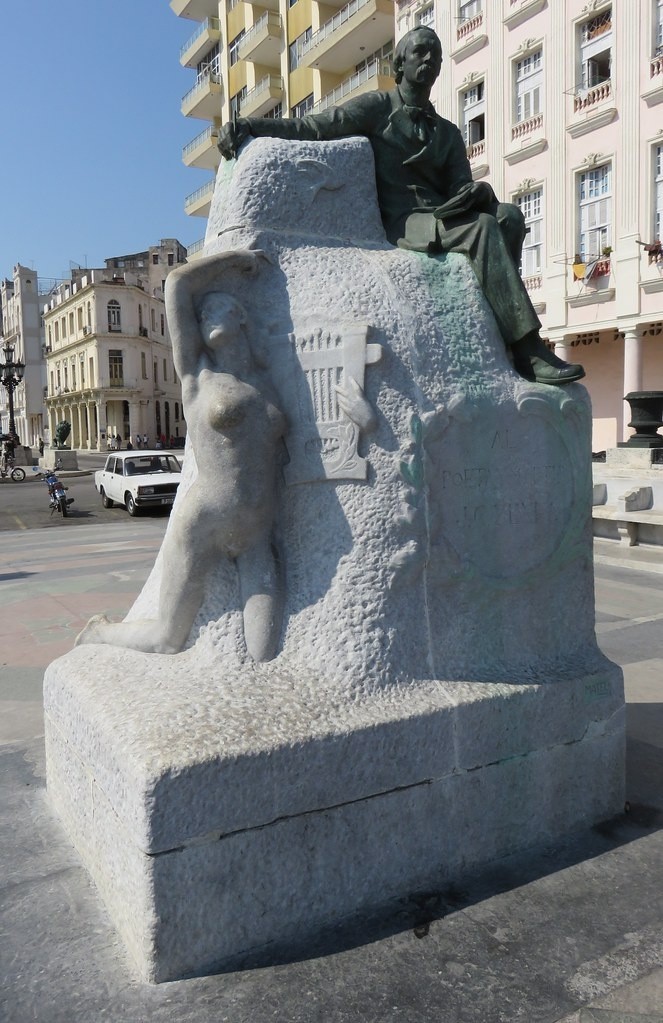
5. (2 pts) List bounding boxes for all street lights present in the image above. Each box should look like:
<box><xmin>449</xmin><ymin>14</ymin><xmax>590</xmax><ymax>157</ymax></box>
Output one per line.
<box><xmin>0</xmin><ymin>342</ymin><xmax>26</xmax><ymax>446</ymax></box>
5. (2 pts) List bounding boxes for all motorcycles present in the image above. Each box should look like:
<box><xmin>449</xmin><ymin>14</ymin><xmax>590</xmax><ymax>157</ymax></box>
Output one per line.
<box><xmin>32</xmin><ymin>458</ymin><xmax>75</xmax><ymax>517</ymax></box>
<box><xmin>0</xmin><ymin>451</ymin><xmax>26</xmax><ymax>482</ymax></box>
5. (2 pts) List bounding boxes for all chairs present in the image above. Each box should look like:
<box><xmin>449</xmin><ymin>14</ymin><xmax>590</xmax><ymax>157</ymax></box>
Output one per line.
<box><xmin>150</xmin><ymin>459</ymin><xmax>166</xmax><ymax>473</ymax></box>
<box><xmin>126</xmin><ymin>461</ymin><xmax>137</xmax><ymax>475</ymax></box>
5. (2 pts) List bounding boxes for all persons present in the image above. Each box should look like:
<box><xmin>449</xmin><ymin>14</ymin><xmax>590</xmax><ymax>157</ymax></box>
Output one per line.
<box><xmin>127</xmin><ymin>442</ymin><xmax>132</xmax><ymax>450</ymax></box>
<box><xmin>74</xmin><ymin>249</ymin><xmax>378</xmax><ymax>659</ymax></box>
<box><xmin>115</xmin><ymin>434</ymin><xmax>122</xmax><ymax>450</ymax></box>
<box><xmin>143</xmin><ymin>433</ymin><xmax>149</xmax><ymax>448</ymax></box>
<box><xmin>136</xmin><ymin>435</ymin><xmax>142</xmax><ymax>449</ymax></box>
<box><xmin>110</xmin><ymin>435</ymin><xmax>116</xmax><ymax>450</ymax></box>
<box><xmin>38</xmin><ymin>438</ymin><xmax>44</xmax><ymax>456</ymax></box>
<box><xmin>154</xmin><ymin>434</ymin><xmax>175</xmax><ymax>449</ymax></box>
<box><xmin>215</xmin><ymin>24</ymin><xmax>586</xmax><ymax>385</ymax></box>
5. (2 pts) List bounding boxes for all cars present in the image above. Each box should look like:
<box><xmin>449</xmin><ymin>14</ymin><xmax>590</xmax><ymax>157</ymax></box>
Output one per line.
<box><xmin>94</xmin><ymin>450</ymin><xmax>183</xmax><ymax>516</ymax></box>
